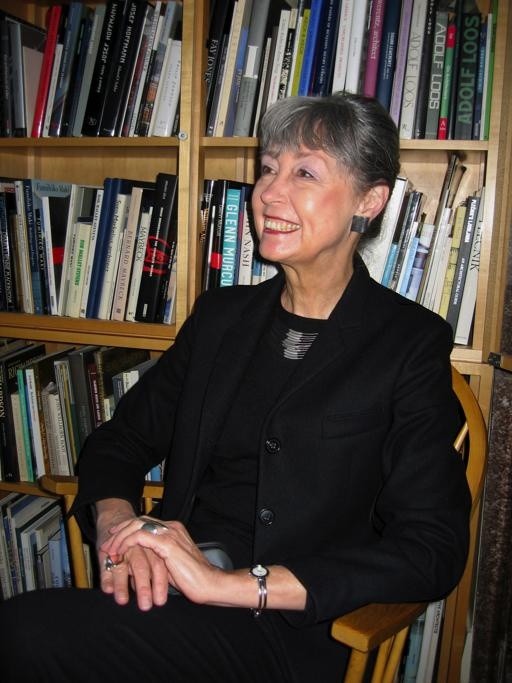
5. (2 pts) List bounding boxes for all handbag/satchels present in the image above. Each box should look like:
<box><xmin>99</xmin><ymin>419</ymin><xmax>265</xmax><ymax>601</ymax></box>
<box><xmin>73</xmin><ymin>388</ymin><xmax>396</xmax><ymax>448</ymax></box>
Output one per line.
<box><xmin>168</xmin><ymin>540</ymin><xmax>236</xmax><ymax>597</ymax></box>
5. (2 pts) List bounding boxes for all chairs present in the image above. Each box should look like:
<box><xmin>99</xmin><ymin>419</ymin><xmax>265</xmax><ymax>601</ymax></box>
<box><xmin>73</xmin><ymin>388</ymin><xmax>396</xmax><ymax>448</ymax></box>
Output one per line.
<box><xmin>40</xmin><ymin>364</ymin><xmax>489</xmax><ymax>681</ymax></box>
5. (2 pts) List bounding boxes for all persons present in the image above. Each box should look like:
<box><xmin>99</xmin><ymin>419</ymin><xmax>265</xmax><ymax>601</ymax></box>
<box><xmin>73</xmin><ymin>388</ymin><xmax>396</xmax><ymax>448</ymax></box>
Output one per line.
<box><xmin>0</xmin><ymin>91</ymin><xmax>470</xmax><ymax>682</ymax></box>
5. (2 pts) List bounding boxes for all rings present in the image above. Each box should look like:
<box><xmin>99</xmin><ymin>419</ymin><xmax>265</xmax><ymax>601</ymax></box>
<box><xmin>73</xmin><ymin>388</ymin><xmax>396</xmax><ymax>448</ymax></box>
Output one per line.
<box><xmin>106</xmin><ymin>557</ymin><xmax>119</xmax><ymax>569</ymax></box>
<box><xmin>142</xmin><ymin>521</ymin><xmax>165</xmax><ymax>534</ymax></box>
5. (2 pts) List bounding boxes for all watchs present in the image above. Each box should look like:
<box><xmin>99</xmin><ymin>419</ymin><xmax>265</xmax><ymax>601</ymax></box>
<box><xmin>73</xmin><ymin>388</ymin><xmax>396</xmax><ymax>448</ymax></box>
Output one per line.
<box><xmin>250</xmin><ymin>564</ymin><xmax>270</xmax><ymax>614</ymax></box>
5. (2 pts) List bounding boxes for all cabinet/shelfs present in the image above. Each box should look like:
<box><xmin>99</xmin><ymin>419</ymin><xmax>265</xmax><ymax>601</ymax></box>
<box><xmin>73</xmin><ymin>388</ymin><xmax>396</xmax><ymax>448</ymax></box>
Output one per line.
<box><xmin>0</xmin><ymin>0</ymin><xmax>512</xmax><ymax>683</ymax></box>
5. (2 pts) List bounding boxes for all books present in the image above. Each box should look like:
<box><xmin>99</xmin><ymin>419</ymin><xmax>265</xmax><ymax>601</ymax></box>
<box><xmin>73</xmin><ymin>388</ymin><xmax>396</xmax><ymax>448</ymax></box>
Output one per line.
<box><xmin>1</xmin><ymin>492</ymin><xmax>98</xmax><ymax>599</ymax></box>
<box><xmin>1</xmin><ymin>0</ymin><xmax>183</xmax><ymax>138</ymax></box>
<box><xmin>357</xmin><ymin>156</ymin><xmax>484</xmax><ymax>345</ymax></box>
<box><xmin>0</xmin><ymin>174</ymin><xmax>178</xmax><ymax>324</ymax></box>
<box><xmin>200</xmin><ymin>181</ymin><xmax>280</xmax><ymax>290</ymax></box>
<box><xmin>0</xmin><ymin>339</ymin><xmax>162</xmax><ymax>481</ymax></box>
<box><xmin>399</xmin><ymin>599</ymin><xmax>445</xmax><ymax>683</ymax></box>
<box><xmin>204</xmin><ymin>1</ymin><xmax>492</xmax><ymax>140</ymax></box>
